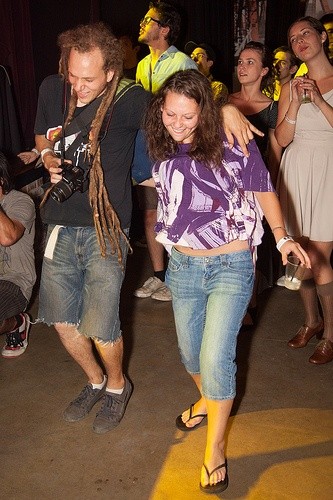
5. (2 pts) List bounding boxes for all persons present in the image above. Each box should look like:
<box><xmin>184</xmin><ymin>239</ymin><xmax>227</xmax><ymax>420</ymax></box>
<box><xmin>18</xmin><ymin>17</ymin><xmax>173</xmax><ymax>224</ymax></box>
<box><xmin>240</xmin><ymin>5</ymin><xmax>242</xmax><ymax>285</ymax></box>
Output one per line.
<box><xmin>34</xmin><ymin>24</ymin><xmax>265</xmax><ymax>435</ymax></box>
<box><xmin>319</xmin><ymin>13</ymin><xmax>333</xmax><ymax>66</ymax></box>
<box><xmin>17</xmin><ymin>146</ymin><xmax>41</xmax><ymax>164</ymax></box>
<box><xmin>142</xmin><ymin>69</ymin><xmax>311</xmax><ymax>494</ymax></box>
<box><xmin>274</xmin><ymin>17</ymin><xmax>333</xmax><ymax>364</ymax></box>
<box><xmin>188</xmin><ymin>40</ymin><xmax>300</xmax><ymax>326</ymax></box>
<box><xmin>0</xmin><ymin>156</ymin><xmax>36</xmax><ymax>359</ymax></box>
<box><xmin>117</xmin><ymin>0</ymin><xmax>198</xmax><ymax>302</ymax></box>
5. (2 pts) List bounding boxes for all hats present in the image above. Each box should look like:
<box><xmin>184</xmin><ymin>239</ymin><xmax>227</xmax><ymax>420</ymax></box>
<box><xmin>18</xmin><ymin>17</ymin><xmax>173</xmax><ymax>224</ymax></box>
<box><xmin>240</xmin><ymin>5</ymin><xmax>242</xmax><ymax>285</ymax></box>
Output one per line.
<box><xmin>184</xmin><ymin>41</ymin><xmax>216</xmax><ymax>66</ymax></box>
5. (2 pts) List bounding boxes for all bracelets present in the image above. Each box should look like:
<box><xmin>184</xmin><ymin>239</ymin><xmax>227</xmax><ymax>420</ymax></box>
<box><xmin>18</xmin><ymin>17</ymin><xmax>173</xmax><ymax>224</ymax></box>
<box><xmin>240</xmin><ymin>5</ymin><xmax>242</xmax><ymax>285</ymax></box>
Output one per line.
<box><xmin>276</xmin><ymin>236</ymin><xmax>295</xmax><ymax>252</ymax></box>
<box><xmin>41</xmin><ymin>148</ymin><xmax>55</xmax><ymax>163</ymax></box>
<box><xmin>272</xmin><ymin>227</ymin><xmax>286</xmax><ymax>234</ymax></box>
<box><xmin>32</xmin><ymin>148</ymin><xmax>40</xmax><ymax>157</ymax></box>
<box><xmin>284</xmin><ymin>114</ymin><xmax>296</xmax><ymax>125</ymax></box>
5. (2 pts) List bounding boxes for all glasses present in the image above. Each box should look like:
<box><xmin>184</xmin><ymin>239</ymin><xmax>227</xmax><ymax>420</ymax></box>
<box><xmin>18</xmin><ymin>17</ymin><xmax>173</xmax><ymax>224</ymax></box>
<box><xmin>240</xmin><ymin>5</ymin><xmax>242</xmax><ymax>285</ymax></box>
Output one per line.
<box><xmin>139</xmin><ymin>15</ymin><xmax>166</xmax><ymax>29</ymax></box>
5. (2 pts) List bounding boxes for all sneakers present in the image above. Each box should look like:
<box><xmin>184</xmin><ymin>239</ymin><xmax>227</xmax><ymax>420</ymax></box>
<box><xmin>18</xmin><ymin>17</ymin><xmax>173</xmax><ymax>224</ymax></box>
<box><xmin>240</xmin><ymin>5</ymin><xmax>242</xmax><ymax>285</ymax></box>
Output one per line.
<box><xmin>134</xmin><ymin>275</ymin><xmax>167</xmax><ymax>298</ymax></box>
<box><xmin>93</xmin><ymin>373</ymin><xmax>134</xmax><ymax>434</ymax></box>
<box><xmin>0</xmin><ymin>313</ymin><xmax>31</xmax><ymax>360</ymax></box>
<box><xmin>150</xmin><ymin>286</ymin><xmax>172</xmax><ymax>301</ymax></box>
<box><xmin>63</xmin><ymin>374</ymin><xmax>108</xmax><ymax>422</ymax></box>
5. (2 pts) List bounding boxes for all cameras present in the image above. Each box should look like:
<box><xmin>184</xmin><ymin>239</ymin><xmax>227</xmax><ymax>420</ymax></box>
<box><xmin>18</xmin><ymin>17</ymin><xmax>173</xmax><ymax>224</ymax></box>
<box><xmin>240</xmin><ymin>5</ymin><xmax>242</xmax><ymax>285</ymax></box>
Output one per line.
<box><xmin>49</xmin><ymin>162</ymin><xmax>90</xmax><ymax>204</ymax></box>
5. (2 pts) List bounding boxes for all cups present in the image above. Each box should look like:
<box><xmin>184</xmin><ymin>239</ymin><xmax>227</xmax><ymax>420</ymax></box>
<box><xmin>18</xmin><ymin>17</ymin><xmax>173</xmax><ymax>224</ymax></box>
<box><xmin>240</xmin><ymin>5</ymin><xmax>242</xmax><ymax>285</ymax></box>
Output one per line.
<box><xmin>297</xmin><ymin>84</ymin><xmax>311</xmax><ymax>104</ymax></box>
<box><xmin>285</xmin><ymin>256</ymin><xmax>306</xmax><ymax>291</ymax></box>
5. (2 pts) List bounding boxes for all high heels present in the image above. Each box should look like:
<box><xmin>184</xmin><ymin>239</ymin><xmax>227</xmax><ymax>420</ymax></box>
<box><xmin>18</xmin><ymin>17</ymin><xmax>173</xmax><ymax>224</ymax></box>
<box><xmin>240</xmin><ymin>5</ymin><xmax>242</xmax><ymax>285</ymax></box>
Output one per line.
<box><xmin>308</xmin><ymin>338</ymin><xmax>333</xmax><ymax>365</ymax></box>
<box><xmin>287</xmin><ymin>318</ymin><xmax>324</xmax><ymax>348</ymax></box>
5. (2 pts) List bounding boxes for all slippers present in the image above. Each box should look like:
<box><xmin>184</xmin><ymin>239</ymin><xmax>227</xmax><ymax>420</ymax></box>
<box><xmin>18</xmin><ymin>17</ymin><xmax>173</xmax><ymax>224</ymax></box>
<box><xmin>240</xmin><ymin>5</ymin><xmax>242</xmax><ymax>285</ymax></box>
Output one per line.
<box><xmin>199</xmin><ymin>457</ymin><xmax>228</xmax><ymax>493</ymax></box>
<box><xmin>175</xmin><ymin>403</ymin><xmax>207</xmax><ymax>431</ymax></box>
